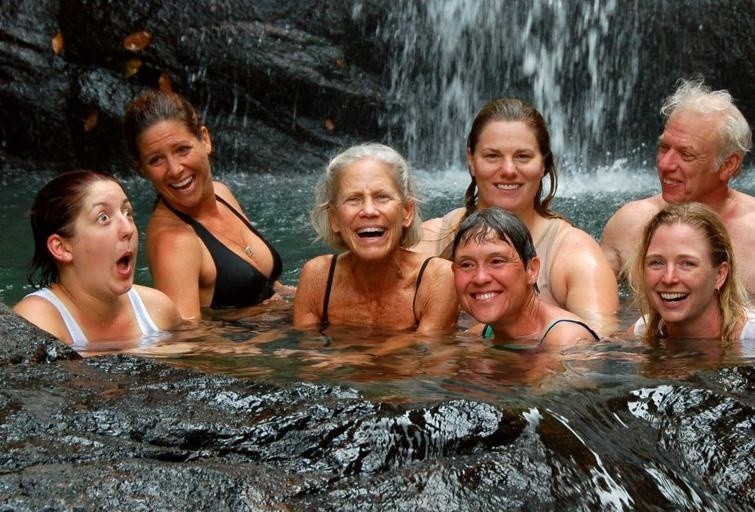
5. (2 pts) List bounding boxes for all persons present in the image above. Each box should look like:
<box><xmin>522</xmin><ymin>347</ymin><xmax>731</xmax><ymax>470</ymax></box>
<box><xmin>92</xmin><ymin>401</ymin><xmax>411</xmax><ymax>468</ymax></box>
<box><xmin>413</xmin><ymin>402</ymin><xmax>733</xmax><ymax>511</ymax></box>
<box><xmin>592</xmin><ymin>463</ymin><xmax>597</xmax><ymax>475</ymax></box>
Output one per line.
<box><xmin>451</xmin><ymin>206</ymin><xmax>601</xmax><ymax>394</ymax></box>
<box><xmin>121</xmin><ymin>92</ymin><xmax>297</xmax><ymax>350</ymax></box>
<box><xmin>608</xmin><ymin>200</ymin><xmax>755</xmax><ymax>379</ymax></box>
<box><xmin>400</xmin><ymin>97</ymin><xmax>620</xmax><ymax>347</ymax></box>
<box><xmin>11</xmin><ymin>168</ymin><xmax>261</xmax><ymax>379</ymax></box>
<box><xmin>292</xmin><ymin>142</ymin><xmax>460</xmax><ymax>383</ymax></box>
<box><xmin>598</xmin><ymin>78</ymin><xmax>755</xmax><ymax>313</ymax></box>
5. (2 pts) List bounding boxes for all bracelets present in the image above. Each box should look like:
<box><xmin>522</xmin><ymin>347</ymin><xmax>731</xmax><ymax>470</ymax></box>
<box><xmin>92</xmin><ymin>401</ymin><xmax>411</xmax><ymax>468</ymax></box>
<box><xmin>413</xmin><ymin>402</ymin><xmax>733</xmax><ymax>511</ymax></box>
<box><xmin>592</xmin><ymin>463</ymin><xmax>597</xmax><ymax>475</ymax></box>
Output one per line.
<box><xmin>363</xmin><ymin>353</ymin><xmax>382</xmax><ymax>362</ymax></box>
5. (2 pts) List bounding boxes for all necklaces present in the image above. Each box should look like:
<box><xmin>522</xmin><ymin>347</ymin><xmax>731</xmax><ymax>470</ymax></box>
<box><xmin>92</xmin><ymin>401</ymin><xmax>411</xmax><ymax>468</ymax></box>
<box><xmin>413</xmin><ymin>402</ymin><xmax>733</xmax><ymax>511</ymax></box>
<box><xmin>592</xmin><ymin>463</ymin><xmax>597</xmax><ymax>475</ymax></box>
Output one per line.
<box><xmin>150</xmin><ymin>204</ymin><xmax>256</xmax><ymax>258</ymax></box>
